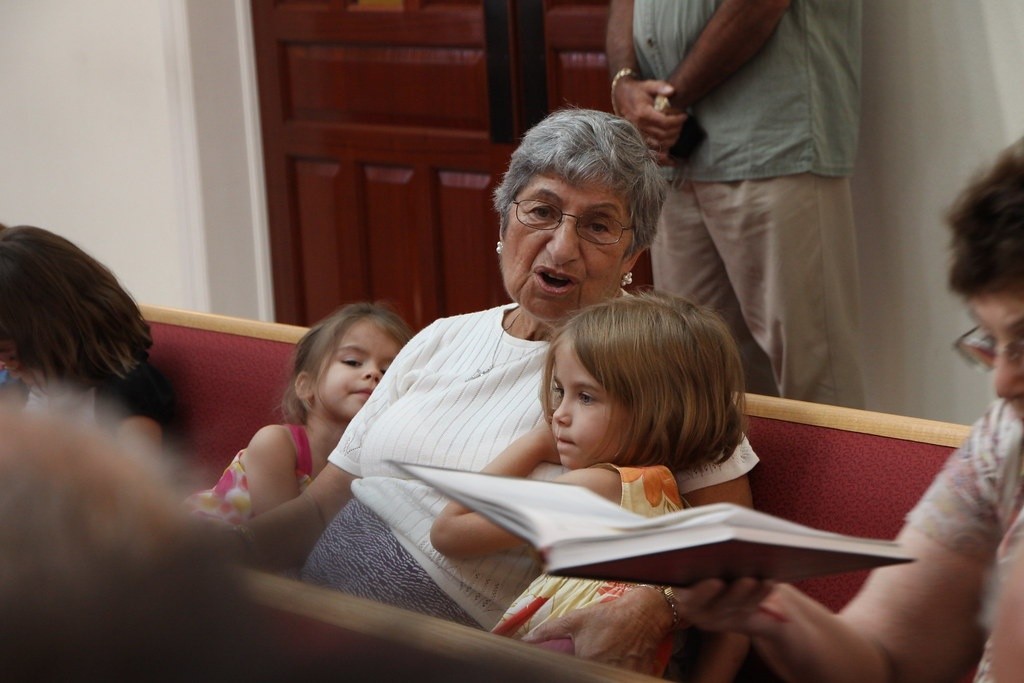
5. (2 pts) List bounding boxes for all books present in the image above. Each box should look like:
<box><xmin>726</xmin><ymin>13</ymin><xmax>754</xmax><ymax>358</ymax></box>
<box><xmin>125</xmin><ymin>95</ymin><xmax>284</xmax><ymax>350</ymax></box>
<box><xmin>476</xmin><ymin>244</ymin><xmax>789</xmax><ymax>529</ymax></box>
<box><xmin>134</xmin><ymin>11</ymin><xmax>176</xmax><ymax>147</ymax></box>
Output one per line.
<box><xmin>391</xmin><ymin>461</ymin><xmax>917</xmax><ymax>587</ymax></box>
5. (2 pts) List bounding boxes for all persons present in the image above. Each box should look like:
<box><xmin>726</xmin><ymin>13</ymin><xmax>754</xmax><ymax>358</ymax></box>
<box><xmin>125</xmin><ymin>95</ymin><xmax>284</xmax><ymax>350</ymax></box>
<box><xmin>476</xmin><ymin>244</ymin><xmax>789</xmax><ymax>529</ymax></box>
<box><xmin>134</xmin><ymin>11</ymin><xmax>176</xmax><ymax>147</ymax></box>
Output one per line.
<box><xmin>432</xmin><ymin>286</ymin><xmax>747</xmax><ymax>677</ymax></box>
<box><xmin>173</xmin><ymin>108</ymin><xmax>760</xmax><ymax>676</ymax></box>
<box><xmin>181</xmin><ymin>298</ymin><xmax>420</xmax><ymax>530</ymax></box>
<box><xmin>0</xmin><ymin>220</ymin><xmax>183</xmax><ymax>683</ymax></box>
<box><xmin>677</xmin><ymin>140</ymin><xmax>1024</xmax><ymax>683</ymax></box>
<box><xmin>605</xmin><ymin>0</ymin><xmax>867</xmax><ymax>408</ymax></box>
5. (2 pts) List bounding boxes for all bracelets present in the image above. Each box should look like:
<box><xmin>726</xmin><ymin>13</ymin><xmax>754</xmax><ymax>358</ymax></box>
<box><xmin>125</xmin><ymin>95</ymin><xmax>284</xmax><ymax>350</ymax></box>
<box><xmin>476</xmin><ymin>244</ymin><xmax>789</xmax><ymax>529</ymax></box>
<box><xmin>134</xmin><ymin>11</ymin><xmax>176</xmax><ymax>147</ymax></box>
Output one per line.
<box><xmin>610</xmin><ymin>67</ymin><xmax>637</xmax><ymax>118</ymax></box>
<box><xmin>641</xmin><ymin>584</ymin><xmax>679</xmax><ymax>609</ymax></box>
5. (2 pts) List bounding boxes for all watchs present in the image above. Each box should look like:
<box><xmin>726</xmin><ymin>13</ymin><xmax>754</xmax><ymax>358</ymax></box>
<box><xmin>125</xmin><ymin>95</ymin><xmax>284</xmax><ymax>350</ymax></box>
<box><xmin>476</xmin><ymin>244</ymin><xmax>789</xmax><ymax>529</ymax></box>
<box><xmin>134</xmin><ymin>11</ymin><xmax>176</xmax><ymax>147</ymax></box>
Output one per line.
<box><xmin>653</xmin><ymin>94</ymin><xmax>672</xmax><ymax>115</ymax></box>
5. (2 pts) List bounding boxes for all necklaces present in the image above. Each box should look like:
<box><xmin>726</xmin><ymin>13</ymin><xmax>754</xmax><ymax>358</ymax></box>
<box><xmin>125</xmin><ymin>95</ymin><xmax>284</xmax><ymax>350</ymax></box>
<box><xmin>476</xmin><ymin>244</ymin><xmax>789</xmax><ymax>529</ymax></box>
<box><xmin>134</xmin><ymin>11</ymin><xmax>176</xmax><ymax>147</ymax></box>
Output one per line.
<box><xmin>465</xmin><ymin>313</ymin><xmax>553</xmax><ymax>383</ymax></box>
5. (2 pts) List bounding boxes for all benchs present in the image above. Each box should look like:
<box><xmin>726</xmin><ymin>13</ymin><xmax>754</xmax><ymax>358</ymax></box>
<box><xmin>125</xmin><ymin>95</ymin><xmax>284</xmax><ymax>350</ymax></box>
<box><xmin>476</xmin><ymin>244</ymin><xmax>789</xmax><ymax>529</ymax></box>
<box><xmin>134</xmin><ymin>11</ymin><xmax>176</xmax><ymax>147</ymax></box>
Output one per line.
<box><xmin>130</xmin><ymin>305</ymin><xmax>966</xmax><ymax>615</ymax></box>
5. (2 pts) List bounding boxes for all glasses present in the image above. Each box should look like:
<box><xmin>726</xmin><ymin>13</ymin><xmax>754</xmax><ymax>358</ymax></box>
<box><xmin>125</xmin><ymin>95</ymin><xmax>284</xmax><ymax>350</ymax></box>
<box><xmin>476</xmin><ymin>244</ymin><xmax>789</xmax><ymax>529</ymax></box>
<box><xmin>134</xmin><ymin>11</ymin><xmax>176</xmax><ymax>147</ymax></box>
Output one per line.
<box><xmin>511</xmin><ymin>199</ymin><xmax>631</xmax><ymax>245</ymax></box>
<box><xmin>953</xmin><ymin>327</ymin><xmax>1024</xmax><ymax>372</ymax></box>
